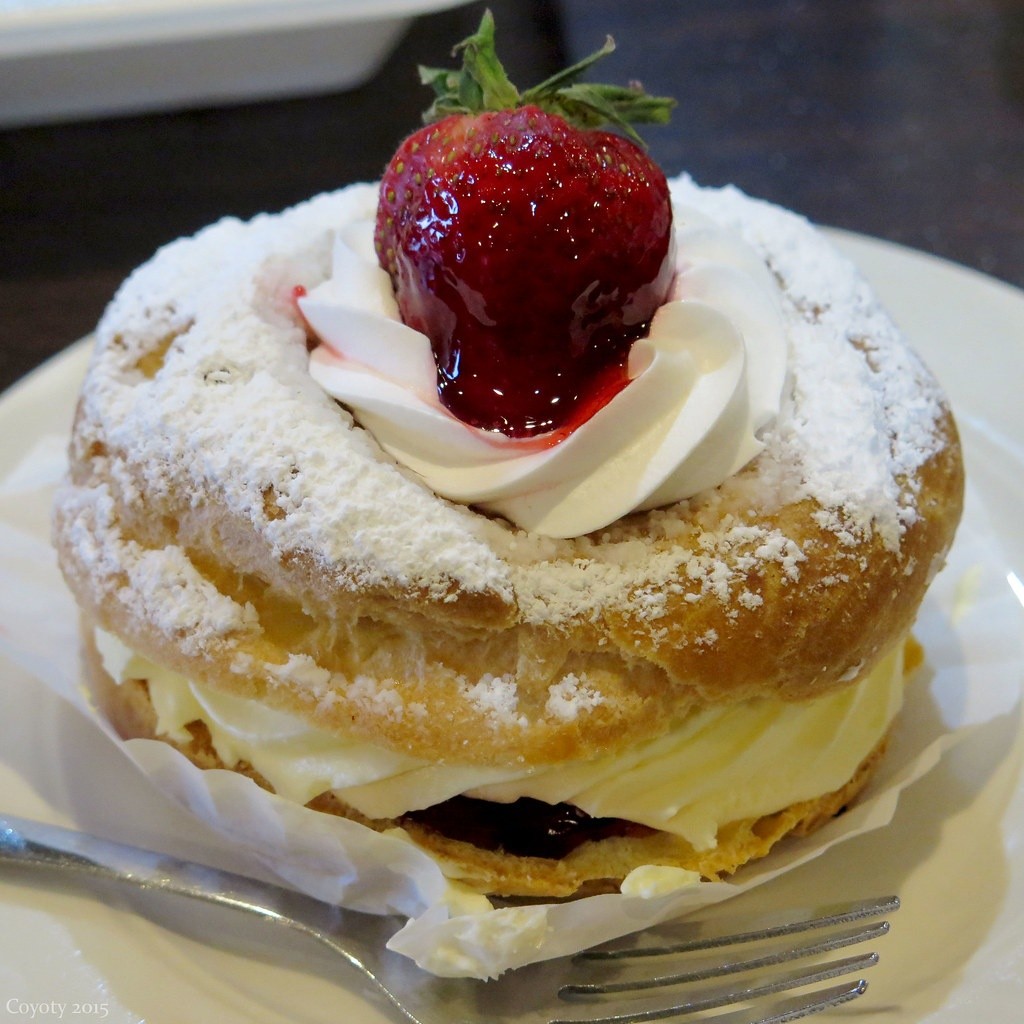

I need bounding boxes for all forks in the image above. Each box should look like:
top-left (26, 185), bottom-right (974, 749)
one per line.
top-left (5, 809), bottom-right (913, 1024)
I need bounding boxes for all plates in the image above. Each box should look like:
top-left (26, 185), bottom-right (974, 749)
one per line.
top-left (0, 216), bottom-right (1024, 1024)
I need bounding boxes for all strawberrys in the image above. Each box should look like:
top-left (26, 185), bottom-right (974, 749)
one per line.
top-left (370, 8), bottom-right (683, 437)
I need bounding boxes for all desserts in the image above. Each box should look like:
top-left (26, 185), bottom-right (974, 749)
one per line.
top-left (58, 174), bottom-right (971, 896)
